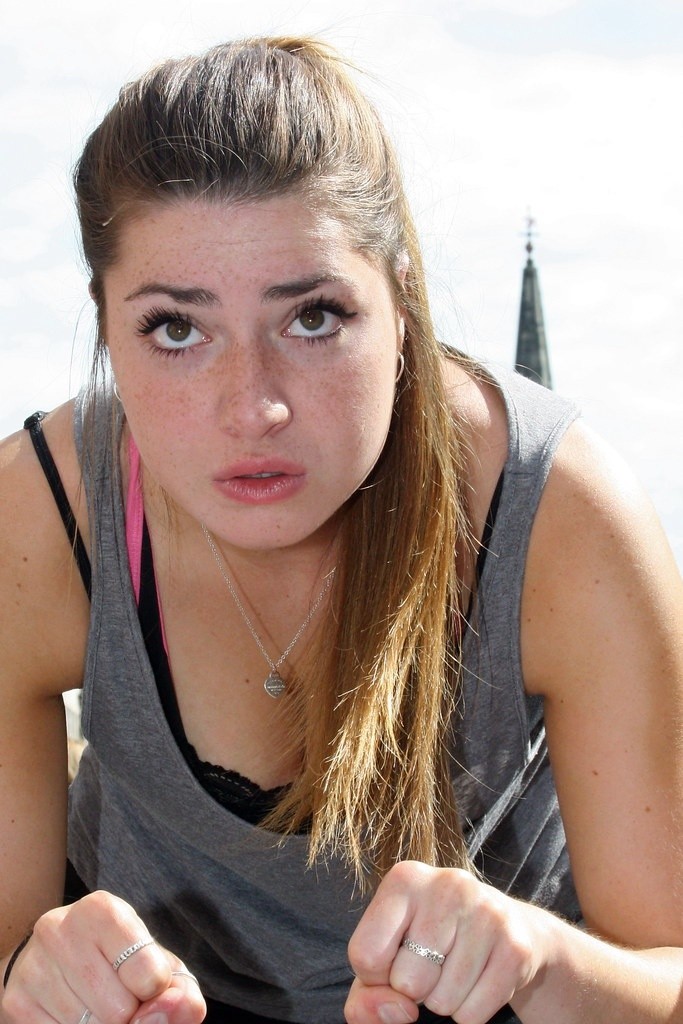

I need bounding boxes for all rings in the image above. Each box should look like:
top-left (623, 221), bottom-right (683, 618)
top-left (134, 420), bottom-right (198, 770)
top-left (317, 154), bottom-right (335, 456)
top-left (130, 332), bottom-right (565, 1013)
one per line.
top-left (400, 936), bottom-right (446, 967)
top-left (80, 1009), bottom-right (91, 1024)
top-left (170, 970), bottom-right (200, 986)
top-left (112, 938), bottom-right (156, 975)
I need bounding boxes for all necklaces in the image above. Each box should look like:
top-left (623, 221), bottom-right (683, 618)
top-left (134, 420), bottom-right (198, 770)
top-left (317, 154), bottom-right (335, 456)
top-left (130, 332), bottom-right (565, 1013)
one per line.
top-left (199, 521), bottom-right (337, 698)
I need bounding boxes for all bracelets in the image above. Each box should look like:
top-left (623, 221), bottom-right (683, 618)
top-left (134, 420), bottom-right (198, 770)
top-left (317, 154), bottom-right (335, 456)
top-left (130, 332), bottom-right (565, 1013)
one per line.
top-left (2, 929), bottom-right (33, 991)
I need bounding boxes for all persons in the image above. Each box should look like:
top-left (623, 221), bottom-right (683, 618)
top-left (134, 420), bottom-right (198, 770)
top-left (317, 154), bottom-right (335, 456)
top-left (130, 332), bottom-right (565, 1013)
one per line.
top-left (0, 35), bottom-right (683, 1024)
